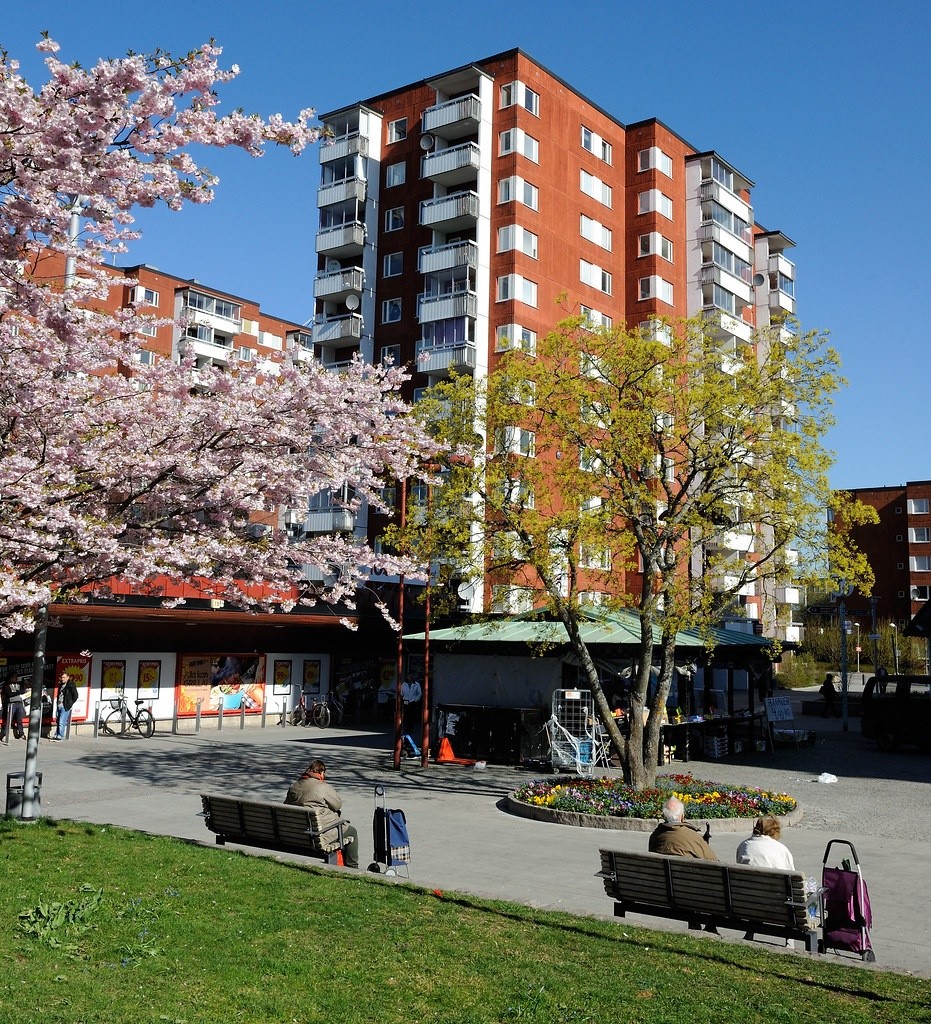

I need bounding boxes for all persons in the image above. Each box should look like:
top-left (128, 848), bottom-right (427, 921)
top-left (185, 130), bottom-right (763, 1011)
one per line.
top-left (402, 673), bottom-right (423, 731)
top-left (735, 814), bottom-right (797, 949)
top-left (49, 672), bottom-right (78, 742)
top-left (648, 796), bottom-right (720, 935)
top-left (283, 760), bottom-right (359, 869)
top-left (876, 665), bottom-right (888, 695)
top-left (2, 672), bottom-right (26, 742)
top-left (821, 674), bottom-right (837, 718)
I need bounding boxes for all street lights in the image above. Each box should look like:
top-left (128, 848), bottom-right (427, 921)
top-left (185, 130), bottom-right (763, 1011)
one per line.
top-left (854, 623), bottom-right (860, 673)
top-left (890, 623), bottom-right (899, 675)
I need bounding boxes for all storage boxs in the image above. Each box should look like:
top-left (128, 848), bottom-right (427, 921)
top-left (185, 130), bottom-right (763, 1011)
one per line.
top-left (704, 728), bottom-right (728, 758)
top-left (754, 739), bottom-right (765, 751)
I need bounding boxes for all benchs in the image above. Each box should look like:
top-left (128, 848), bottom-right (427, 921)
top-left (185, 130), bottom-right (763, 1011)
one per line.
top-left (594, 847), bottom-right (831, 955)
top-left (195, 792), bottom-right (354, 864)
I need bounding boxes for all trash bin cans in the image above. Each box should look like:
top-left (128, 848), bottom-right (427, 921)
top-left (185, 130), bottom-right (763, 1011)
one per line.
top-left (7, 772), bottom-right (42, 818)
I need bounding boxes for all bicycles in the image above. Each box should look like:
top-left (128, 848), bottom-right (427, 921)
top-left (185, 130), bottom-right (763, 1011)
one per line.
top-left (291, 684), bottom-right (330, 729)
top-left (105, 693), bottom-right (155, 738)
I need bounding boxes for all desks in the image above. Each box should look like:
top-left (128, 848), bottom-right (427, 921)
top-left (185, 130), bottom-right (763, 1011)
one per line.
top-left (658, 709), bottom-right (766, 766)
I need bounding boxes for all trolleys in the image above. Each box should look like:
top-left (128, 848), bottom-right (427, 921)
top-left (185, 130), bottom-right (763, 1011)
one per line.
top-left (550, 688), bottom-right (596, 774)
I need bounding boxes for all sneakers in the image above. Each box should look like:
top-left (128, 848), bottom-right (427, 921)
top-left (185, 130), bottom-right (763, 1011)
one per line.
top-left (50, 737), bottom-right (61, 742)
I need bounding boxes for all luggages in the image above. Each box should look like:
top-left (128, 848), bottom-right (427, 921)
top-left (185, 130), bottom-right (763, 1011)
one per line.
top-left (817, 839), bottom-right (876, 962)
top-left (367, 784), bottom-right (412, 879)
